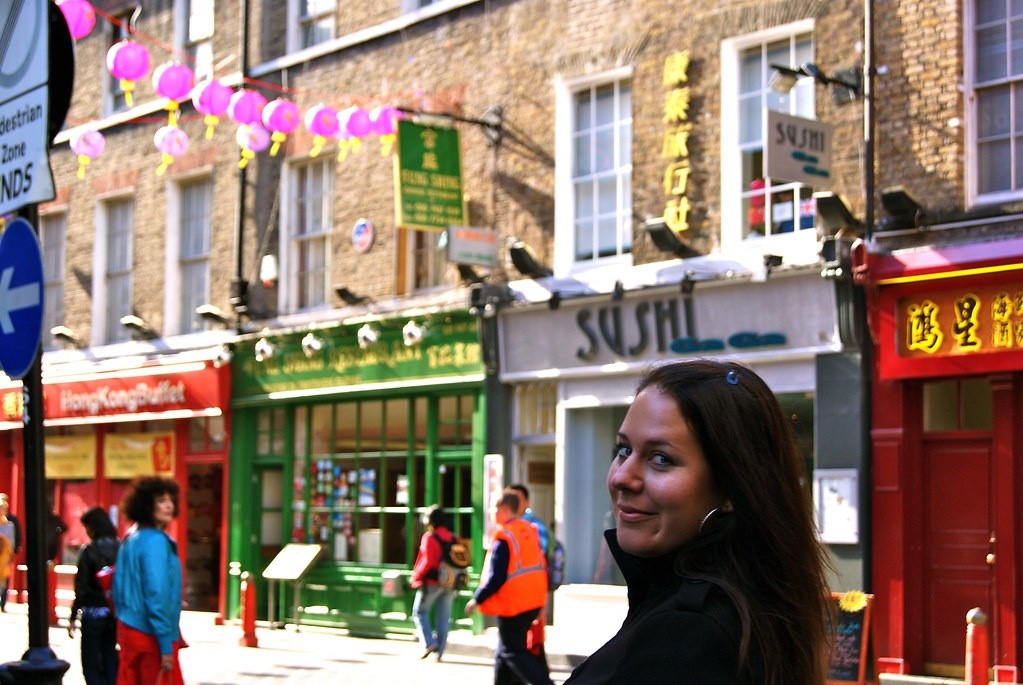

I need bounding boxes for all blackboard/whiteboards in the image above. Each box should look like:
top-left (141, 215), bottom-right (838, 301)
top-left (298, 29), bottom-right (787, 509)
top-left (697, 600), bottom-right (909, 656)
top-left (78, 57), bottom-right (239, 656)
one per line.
top-left (823, 591), bottom-right (880, 685)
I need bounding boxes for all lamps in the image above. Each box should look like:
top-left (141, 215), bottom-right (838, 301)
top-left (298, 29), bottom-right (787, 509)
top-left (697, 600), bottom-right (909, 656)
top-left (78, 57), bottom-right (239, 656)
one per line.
top-left (300, 333), bottom-right (325, 359)
top-left (880, 187), bottom-right (925, 228)
top-left (402, 318), bottom-right (429, 346)
top-left (357, 323), bottom-right (383, 349)
top-left (458, 263), bottom-right (488, 288)
top-left (49, 325), bottom-right (89, 348)
top-left (120, 314), bottom-right (158, 340)
top-left (643, 219), bottom-right (702, 259)
top-left (195, 303), bottom-right (231, 329)
top-left (254, 339), bottom-right (280, 364)
top-left (816, 190), bottom-right (862, 234)
top-left (508, 244), bottom-right (553, 279)
top-left (337, 288), bottom-right (369, 306)
top-left (766, 62), bottom-right (862, 101)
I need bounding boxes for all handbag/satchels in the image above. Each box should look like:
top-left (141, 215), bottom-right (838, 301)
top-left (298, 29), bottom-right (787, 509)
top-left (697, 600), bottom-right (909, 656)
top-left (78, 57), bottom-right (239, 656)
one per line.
top-left (97, 561), bottom-right (117, 613)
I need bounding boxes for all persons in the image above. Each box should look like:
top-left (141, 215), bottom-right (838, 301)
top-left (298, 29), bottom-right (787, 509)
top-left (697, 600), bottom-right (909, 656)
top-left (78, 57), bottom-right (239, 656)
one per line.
top-left (47, 499), bottom-right (67, 626)
top-left (111, 476), bottom-right (189, 685)
top-left (408, 506), bottom-right (453, 661)
top-left (67, 507), bottom-right (120, 685)
top-left (563, 360), bottom-right (834, 685)
top-left (0, 493), bottom-right (22, 613)
top-left (464, 485), bottom-right (556, 685)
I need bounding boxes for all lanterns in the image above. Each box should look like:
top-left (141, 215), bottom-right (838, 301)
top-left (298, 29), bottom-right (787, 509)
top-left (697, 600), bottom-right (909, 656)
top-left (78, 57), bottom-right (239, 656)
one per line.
top-left (304, 103), bottom-right (402, 162)
top-left (234, 125), bottom-right (269, 168)
top-left (153, 126), bottom-right (190, 177)
top-left (69, 127), bottom-right (105, 179)
top-left (106, 38), bottom-right (150, 105)
top-left (54, 0), bottom-right (96, 39)
top-left (191, 79), bottom-right (300, 156)
top-left (151, 60), bottom-right (195, 126)
top-left (748, 177), bottom-right (766, 208)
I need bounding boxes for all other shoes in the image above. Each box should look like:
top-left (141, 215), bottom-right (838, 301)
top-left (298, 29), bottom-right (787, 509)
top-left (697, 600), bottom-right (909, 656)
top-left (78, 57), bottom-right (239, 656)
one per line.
top-left (0, 605), bottom-right (7, 613)
top-left (436, 656), bottom-right (442, 661)
top-left (421, 642), bottom-right (439, 658)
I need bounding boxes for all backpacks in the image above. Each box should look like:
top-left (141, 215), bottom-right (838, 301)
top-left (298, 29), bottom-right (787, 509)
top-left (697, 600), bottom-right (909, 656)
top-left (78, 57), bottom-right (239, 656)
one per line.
top-left (548, 529), bottom-right (564, 592)
top-left (429, 531), bottom-right (469, 589)
